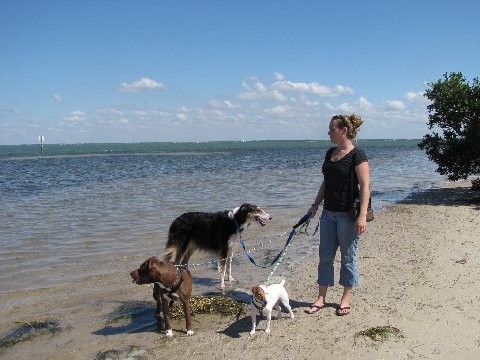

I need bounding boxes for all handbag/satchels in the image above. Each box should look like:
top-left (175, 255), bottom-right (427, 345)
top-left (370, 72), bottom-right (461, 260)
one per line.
top-left (351, 203), bottom-right (374, 222)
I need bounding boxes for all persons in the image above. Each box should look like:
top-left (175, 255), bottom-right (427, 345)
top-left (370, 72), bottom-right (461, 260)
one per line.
top-left (304, 114), bottom-right (370, 316)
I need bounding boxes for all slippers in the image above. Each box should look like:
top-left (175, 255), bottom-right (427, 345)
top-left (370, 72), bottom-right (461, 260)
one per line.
top-left (336, 304), bottom-right (351, 316)
top-left (303, 302), bottom-right (325, 313)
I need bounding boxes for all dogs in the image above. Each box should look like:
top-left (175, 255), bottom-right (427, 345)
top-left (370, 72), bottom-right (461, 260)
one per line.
top-left (161, 202), bottom-right (274, 290)
top-left (129, 257), bottom-right (196, 339)
top-left (249, 278), bottom-right (295, 335)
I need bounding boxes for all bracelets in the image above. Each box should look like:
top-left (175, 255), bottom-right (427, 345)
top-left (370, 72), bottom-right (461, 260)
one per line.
top-left (312, 203), bottom-right (318, 206)
